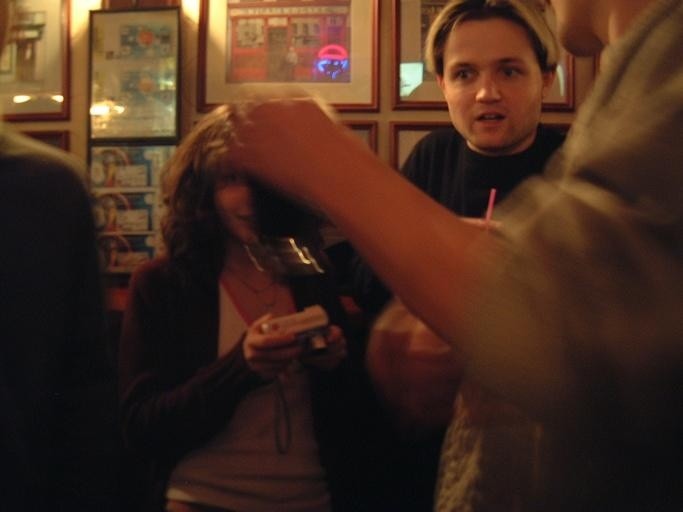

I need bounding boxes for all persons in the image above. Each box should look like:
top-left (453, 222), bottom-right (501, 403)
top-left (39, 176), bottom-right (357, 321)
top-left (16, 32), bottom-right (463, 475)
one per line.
top-left (2, 0), bottom-right (681, 511)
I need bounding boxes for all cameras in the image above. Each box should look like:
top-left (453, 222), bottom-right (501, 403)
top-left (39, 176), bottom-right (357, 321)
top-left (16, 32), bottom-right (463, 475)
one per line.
top-left (259, 305), bottom-right (331, 371)
